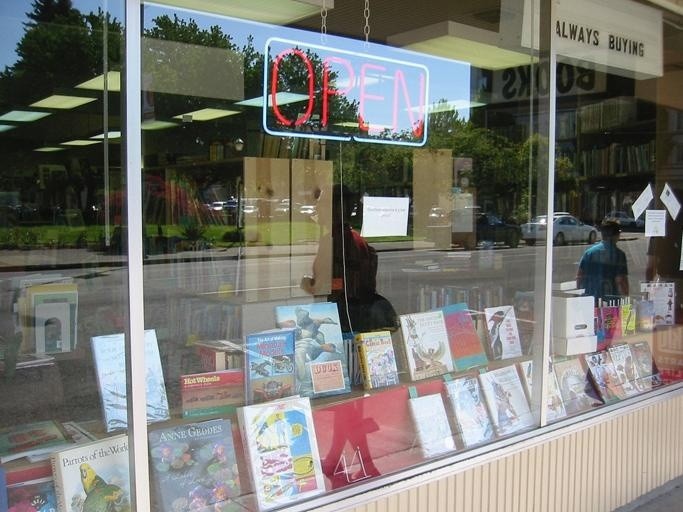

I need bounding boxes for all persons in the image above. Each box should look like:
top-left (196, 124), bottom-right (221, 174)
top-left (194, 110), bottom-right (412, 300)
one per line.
top-left (306, 182), bottom-right (380, 481)
top-left (575, 216), bottom-right (631, 405)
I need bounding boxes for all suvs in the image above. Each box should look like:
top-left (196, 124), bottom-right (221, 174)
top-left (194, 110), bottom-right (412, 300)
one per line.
top-left (422, 207), bottom-right (522, 251)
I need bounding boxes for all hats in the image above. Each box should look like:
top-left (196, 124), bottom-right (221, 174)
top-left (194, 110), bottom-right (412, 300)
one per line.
top-left (332, 183), bottom-right (361, 199)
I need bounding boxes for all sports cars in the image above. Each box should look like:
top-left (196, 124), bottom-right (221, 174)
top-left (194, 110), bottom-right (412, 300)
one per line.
top-left (252, 380), bottom-right (291, 404)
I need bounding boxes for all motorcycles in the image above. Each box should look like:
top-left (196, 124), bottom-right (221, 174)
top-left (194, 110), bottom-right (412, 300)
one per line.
top-left (274, 355), bottom-right (292, 373)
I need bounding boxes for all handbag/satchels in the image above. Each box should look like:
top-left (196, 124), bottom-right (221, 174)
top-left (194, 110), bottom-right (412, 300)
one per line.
top-left (352, 288), bottom-right (402, 339)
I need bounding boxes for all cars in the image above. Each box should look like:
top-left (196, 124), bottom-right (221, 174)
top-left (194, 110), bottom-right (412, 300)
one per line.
top-left (520, 212), bottom-right (598, 247)
top-left (203, 196), bottom-right (481, 226)
top-left (602, 210), bottom-right (644, 231)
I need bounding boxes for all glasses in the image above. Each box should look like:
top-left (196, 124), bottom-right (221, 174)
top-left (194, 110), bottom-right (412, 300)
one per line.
top-left (618, 227), bottom-right (623, 233)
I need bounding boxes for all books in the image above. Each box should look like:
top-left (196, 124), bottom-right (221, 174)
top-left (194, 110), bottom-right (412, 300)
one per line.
top-left (1, 278), bottom-right (326, 510)
top-left (275, 277), bottom-right (567, 460)
top-left (553, 279), bottom-right (681, 416)
top-left (556, 98), bottom-right (656, 224)
